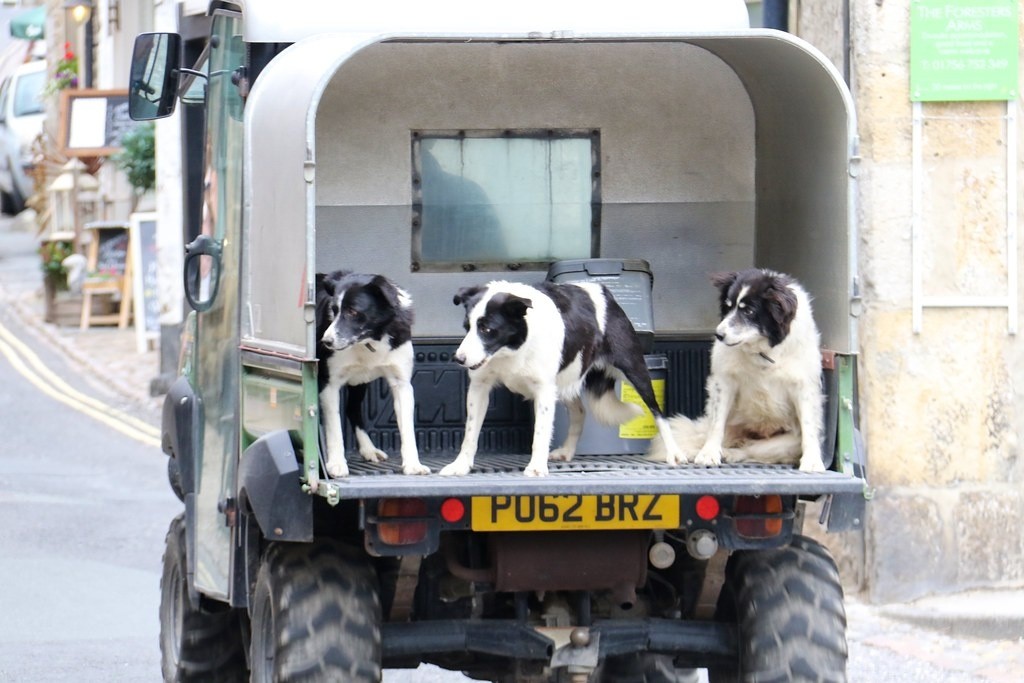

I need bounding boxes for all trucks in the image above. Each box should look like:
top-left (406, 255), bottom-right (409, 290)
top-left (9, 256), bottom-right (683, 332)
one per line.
top-left (126, 0), bottom-right (870, 683)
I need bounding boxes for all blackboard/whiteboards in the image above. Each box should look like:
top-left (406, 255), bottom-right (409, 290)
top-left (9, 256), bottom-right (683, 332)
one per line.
top-left (132, 211), bottom-right (166, 340)
top-left (82, 225), bottom-right (134, 290)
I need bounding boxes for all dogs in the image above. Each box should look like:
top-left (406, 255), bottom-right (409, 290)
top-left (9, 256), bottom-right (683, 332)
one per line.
top-left (314, 270), bottom-right (432, 477)
top-left (650, 268), bottom-right (828, 474)
top-left (433, 279), bottom-right (690, 477)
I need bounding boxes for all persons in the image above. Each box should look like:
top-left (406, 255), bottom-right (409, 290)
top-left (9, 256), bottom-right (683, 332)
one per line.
top-left (419, 133), bottom-right (506, 263)
top-left (129, 33), bottom-right (157, 92)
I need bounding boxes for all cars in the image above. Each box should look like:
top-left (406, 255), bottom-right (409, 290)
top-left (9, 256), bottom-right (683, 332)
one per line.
top-left (0, 61), bottom-right (48, 215)
